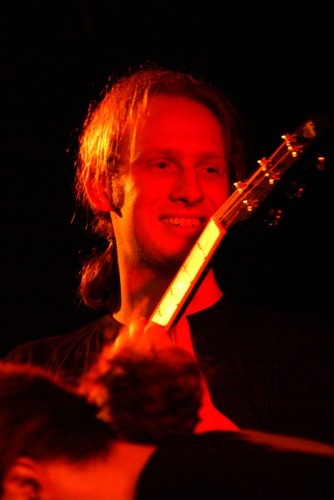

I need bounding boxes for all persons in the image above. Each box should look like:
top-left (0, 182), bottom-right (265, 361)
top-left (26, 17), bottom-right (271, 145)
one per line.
top-left (0, 360), bottom-right (334, 500)
top-left (0, 67), bottom-right (334, 457)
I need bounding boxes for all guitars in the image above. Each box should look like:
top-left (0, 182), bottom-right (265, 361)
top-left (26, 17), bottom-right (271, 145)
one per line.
top-left (150, 116), bottom-right (327, 334)
